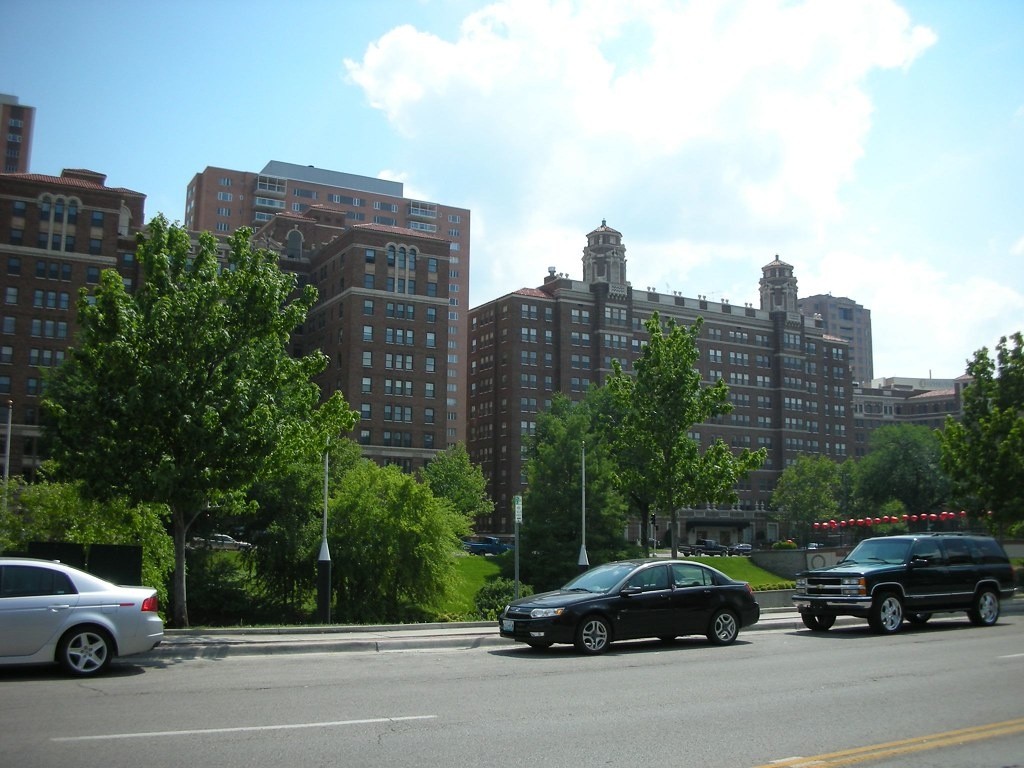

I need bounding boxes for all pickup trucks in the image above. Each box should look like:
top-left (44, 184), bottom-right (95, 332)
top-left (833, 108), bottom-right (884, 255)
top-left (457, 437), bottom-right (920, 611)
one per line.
top-left (678, 538), bottom-right (728, 557)
top-left (463, 537), bottom-right (514, 556)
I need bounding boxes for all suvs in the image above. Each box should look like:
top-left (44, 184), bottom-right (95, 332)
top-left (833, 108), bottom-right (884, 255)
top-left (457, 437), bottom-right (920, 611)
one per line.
top-left (791, 532), bottom-right (1017, 635)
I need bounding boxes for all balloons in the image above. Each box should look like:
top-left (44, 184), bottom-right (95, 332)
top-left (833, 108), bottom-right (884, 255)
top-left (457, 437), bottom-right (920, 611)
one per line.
top-left (988, 511), bottom-right (992, 515)
top-left (813, 511), bottom-right (966, 528)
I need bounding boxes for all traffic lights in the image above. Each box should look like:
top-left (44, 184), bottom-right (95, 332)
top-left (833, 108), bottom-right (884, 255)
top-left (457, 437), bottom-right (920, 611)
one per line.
top-left (650, 513), bottom-right (655, 524)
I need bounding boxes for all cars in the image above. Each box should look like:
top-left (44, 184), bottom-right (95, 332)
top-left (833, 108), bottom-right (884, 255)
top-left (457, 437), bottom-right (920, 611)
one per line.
top-left (802, 542), bottom-right (824, 549)
top-left (727, 543), bottom-right (751, 556)
top-left (498, 558), bottom-right (760, 655)
top-left (191, 534), bottom-right (251, 551)
top-left (0, 556), bottom-right (164, 678)
